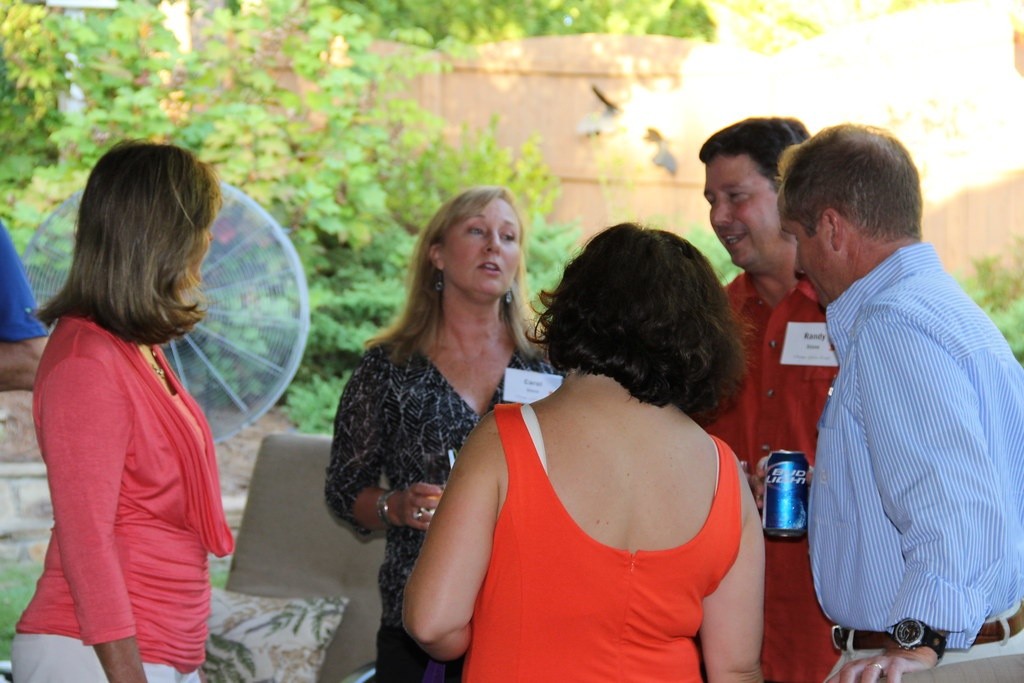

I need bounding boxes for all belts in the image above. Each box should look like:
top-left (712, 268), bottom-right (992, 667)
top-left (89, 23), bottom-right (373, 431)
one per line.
top-left (834, 596), bottom-right (1023, 650)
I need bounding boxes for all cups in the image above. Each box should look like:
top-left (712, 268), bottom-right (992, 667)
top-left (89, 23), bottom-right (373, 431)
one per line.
top-left (418, 449), bottom-right (456, 520)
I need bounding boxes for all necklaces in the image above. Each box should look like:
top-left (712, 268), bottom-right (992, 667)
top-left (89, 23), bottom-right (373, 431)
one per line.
top-left (138, 343), bottom-right (164, 375)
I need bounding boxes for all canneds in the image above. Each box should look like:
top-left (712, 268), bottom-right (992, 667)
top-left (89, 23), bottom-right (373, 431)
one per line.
top-left (761, 449), bottom-right (809, 537)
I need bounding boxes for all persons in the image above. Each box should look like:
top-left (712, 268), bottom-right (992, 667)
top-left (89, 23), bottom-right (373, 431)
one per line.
top-left (0, 220), bottom-right (50, 392)
top-left (692, 116), bottom-right (842, 683)
top-left (401, 223), bottom-right (766, 683)
top-left (324, 186), bottom-right (578, 683)
top-left (11, 139), bottom-right (235, 683)
top-left (750, 123), bottom-right (1024, 683)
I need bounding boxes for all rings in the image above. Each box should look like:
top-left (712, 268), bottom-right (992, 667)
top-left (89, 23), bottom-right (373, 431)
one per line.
top-left (867, 663), bottom-right (883, 678)
top-left (417, 508), bottom-right (423, 520)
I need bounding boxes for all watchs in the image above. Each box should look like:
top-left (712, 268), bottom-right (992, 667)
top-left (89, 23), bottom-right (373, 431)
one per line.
top-left (376, 490), bottom-right (404, 528)
top-left (891, 618), bottom-right (947, 658)
